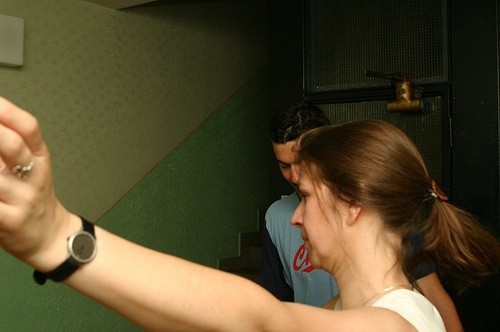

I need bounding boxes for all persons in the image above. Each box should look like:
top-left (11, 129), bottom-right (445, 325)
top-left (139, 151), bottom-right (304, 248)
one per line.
top-left (264, 99), bottom-right (466, 332)
top-left (0, 97), bottom-right (500, 332)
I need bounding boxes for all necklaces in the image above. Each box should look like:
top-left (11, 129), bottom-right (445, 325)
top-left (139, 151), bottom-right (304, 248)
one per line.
top-left (363, 284), bottom-right (412, 306)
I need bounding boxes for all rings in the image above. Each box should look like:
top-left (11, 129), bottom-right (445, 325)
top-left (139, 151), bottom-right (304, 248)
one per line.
top-left (13, 161), bottom-right (34, 178)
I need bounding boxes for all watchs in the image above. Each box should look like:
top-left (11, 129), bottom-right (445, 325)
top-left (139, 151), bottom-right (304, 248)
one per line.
top-left (33, 216), bottom-right (97, 285)
